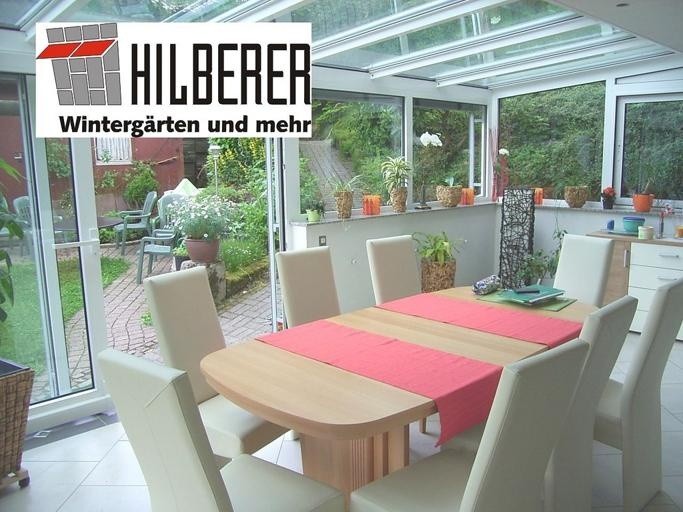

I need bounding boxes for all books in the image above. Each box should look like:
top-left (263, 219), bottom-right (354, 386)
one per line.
top-left (499, 284), bottom-right (566, 306)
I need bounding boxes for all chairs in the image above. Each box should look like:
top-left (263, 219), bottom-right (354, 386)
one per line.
top-left (117, 190), bottom-right (181, 283)
top-left (12, 191), bottom-right (63, 261)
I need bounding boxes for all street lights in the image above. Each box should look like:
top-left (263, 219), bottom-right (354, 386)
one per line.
top-left (209, 143), bottom-right (221, 203)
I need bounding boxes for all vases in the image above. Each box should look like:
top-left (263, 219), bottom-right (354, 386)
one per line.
top-left (182, 236), bottom-right (221, 264)
top-left (301, 156), bottom-right (474, 223)
top-left (526, 184), bottom-right (667, 241)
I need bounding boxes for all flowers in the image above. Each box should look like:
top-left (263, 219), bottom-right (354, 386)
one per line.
top-left (161, 192), bottom-right (246, 239)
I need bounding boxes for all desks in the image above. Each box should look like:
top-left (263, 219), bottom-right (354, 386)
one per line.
top-left (50, 212), bottom-right (124, 238)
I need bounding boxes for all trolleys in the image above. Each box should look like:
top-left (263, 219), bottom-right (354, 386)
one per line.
top-left (0, 467), bottom-right (30, 493)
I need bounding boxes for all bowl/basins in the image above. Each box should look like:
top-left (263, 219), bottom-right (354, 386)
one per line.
top-left (623, 216), bottom-right (644, 231)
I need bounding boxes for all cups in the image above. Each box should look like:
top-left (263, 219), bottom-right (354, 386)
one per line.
top-left (530, 188), bottom-right (543, 205)
top-left (362, 194), bottom-right (379, 215)
top-left (675, 226), bottom-right (682, 238)
top-left (637, 226), bottom-right (653, 239)
top-left (458, 189), bottom-right (474, 205)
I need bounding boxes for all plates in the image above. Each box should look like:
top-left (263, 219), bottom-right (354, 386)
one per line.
top-left (610, 230), bottom-right (638, 236)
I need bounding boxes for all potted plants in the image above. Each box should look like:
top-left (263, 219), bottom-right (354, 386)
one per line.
top-left (0, 155), bottom-right (35, 491)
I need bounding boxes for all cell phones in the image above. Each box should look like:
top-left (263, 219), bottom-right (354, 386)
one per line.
top-left (515, 288), bottom-right (539, 293)
